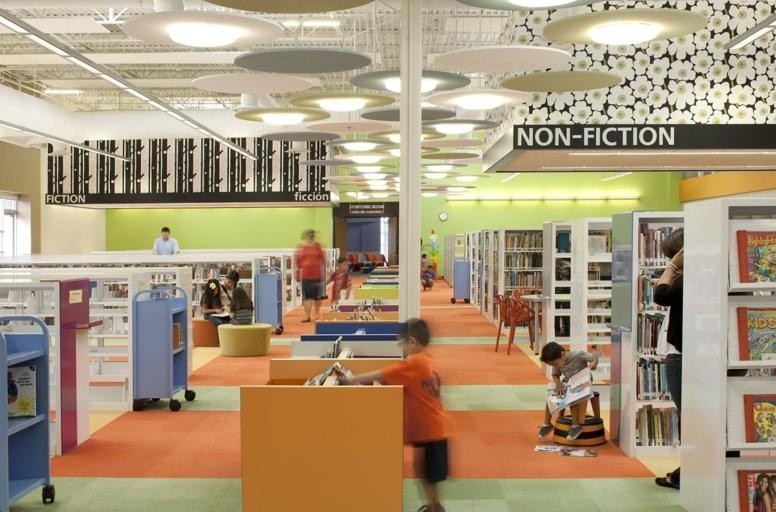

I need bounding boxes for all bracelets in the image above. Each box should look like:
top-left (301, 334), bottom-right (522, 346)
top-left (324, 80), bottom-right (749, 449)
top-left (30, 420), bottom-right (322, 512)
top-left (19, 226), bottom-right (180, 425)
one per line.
top-left (668, 261), bottom-right (681, 274)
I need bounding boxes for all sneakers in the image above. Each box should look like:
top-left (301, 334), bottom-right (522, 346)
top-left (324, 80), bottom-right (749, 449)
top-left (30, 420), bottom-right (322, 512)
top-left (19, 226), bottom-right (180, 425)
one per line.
top-left (537, 423), bottom-right (553, 439)
top-left (566, 424), bottom-right (585, 441)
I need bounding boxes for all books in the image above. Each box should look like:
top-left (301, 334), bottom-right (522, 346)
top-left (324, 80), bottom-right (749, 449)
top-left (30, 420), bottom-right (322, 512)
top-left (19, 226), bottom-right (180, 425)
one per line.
top-left (637, 224), bottom-right (683, 447)
top-left (535, 444), bottom-right (596, 457)
top-left (95, 255), bottom-right (301, 326)
top-left (742, 392), bottom-right (776, 443)
top-left (465, 231), bottom-right (544, 326)
top-left (738, 469), bottom-right (776, 511)
top-left (7, 362), bottom-right (39, 419)
top-left (736, 305), bottom-right (776, 360)
top-left (736, 228), bottom-right (776, 284)
top-left (300, 336), bottom-right (356, 385)
top-left (547, 366), bottom-right (595, 417)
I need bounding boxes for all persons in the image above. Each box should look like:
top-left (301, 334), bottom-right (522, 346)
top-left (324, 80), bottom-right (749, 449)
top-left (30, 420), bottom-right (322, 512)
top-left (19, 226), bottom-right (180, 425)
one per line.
top-left (296, 229), bottom-right (328, 324)
top-left (339, 317), bottom-right (451, 512)
top-left (422, 254), bottom-right (428, 270)
top-left (420, 265), bottom-right (435, 291)
top-left (326, 255), bottom-right (352, 309)
top-left (752, 473), bottom-right (775, 511)
top-left (654, 227), bottom-right (684, 489)
top-left (536, 342), bottom-right (599, 443)
top-left (153, 226), bottom-right (180, 256)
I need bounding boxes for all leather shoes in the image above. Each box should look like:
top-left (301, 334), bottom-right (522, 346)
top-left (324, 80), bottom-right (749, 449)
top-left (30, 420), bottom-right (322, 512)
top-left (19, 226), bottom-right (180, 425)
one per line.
top-left (301, 318), bottom-right (311, 322)
top-left (655, 472), bottom-right (679, 490)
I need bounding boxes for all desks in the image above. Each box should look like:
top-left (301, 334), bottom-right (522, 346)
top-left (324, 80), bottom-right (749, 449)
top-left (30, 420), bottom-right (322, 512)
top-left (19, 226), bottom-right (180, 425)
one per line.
top-left (243, 271), bottom-right (405, 512)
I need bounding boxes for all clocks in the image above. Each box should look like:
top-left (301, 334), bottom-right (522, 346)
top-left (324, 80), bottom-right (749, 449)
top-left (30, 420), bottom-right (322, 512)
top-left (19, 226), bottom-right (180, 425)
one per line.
top-left (439, 211), bottom-right (448, 221)
